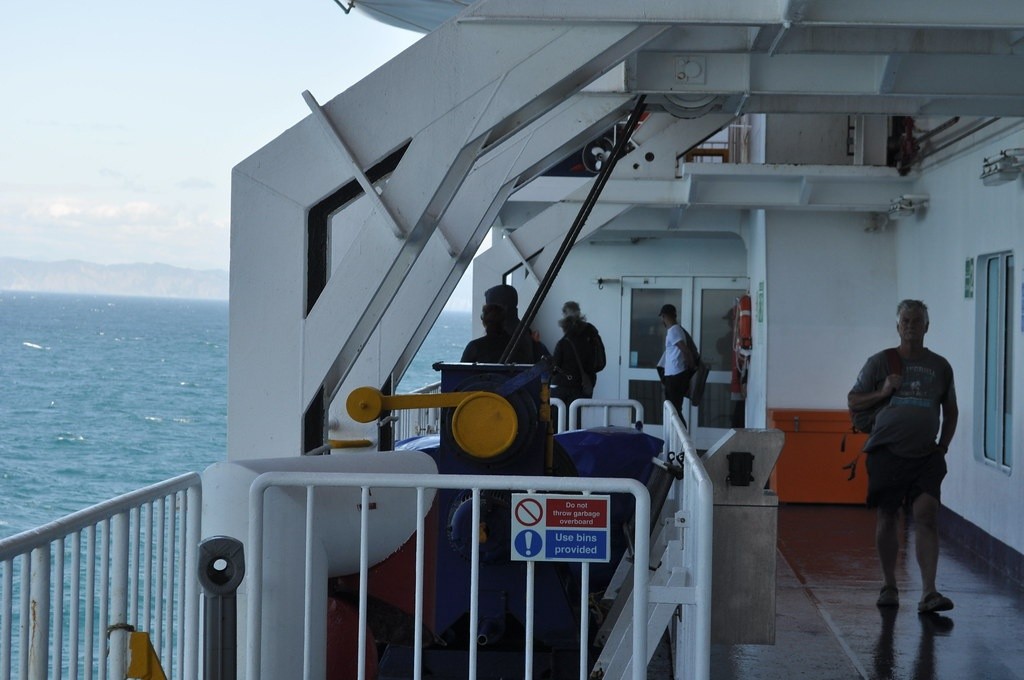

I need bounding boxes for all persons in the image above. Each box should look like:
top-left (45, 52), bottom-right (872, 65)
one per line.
top-left (657, 304), bottom-right (701, 428)
top-left (461, 284), bottom-right (552, 365)
top-left (551, 300), bottom-right (607, 399)
top-left (847, 299), bottom-right (959, 611)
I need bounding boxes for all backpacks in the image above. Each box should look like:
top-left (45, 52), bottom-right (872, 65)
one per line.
top-left (588, 333), bottom-right (607, 374)
top-left (851, 348), bottom-right (903, 434)
top-left (548, 368), bottom-right (584, 405)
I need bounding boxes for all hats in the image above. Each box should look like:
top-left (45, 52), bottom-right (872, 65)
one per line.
top-left (658, 303), bottom-right (676, 317)
top-left (486, 284), bottom-right (519, 309)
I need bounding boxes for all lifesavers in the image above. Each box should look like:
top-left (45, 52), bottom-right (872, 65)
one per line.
top-left (733, 294), bottom-right (752, 361)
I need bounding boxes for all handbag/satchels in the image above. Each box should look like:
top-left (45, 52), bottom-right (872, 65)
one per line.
top-left (684, 367), bottom-right (711, 406)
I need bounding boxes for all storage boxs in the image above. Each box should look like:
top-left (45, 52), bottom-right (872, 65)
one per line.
top-left (767, 405), bottom-right (871, 504)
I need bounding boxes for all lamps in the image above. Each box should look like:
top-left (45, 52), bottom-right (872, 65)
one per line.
top-left (977, 147), bottom-right (1024, 187)
top-left (886, 192), bottom-right (928, 221)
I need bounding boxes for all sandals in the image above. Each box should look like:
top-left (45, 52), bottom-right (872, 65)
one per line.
top-left (918, 592), bottom-right (954, 612)
top-left (876, 584), bottom-right (900, 605)
